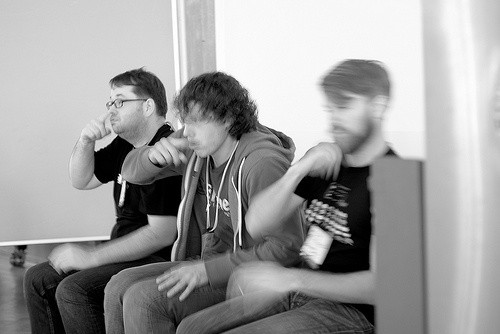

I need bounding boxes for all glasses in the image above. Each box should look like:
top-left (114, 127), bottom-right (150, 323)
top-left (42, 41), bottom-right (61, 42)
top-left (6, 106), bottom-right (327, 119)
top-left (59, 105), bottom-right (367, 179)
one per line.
top-left (106, 99), bottom-right (147, 110)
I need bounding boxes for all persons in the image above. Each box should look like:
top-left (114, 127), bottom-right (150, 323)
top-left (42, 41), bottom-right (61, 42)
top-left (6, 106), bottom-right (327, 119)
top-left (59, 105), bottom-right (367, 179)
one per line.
top-left (23, 68), bottom-right (202, 334)
top-left (176, 60), bottom-right (402, 334)
top-left (103, 72), bottom-right (306, 334)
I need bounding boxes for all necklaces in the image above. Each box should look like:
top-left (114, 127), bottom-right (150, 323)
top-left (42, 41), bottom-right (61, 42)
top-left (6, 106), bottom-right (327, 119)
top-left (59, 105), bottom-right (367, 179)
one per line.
top-left (117, 122), bottom-right (176, 184)
